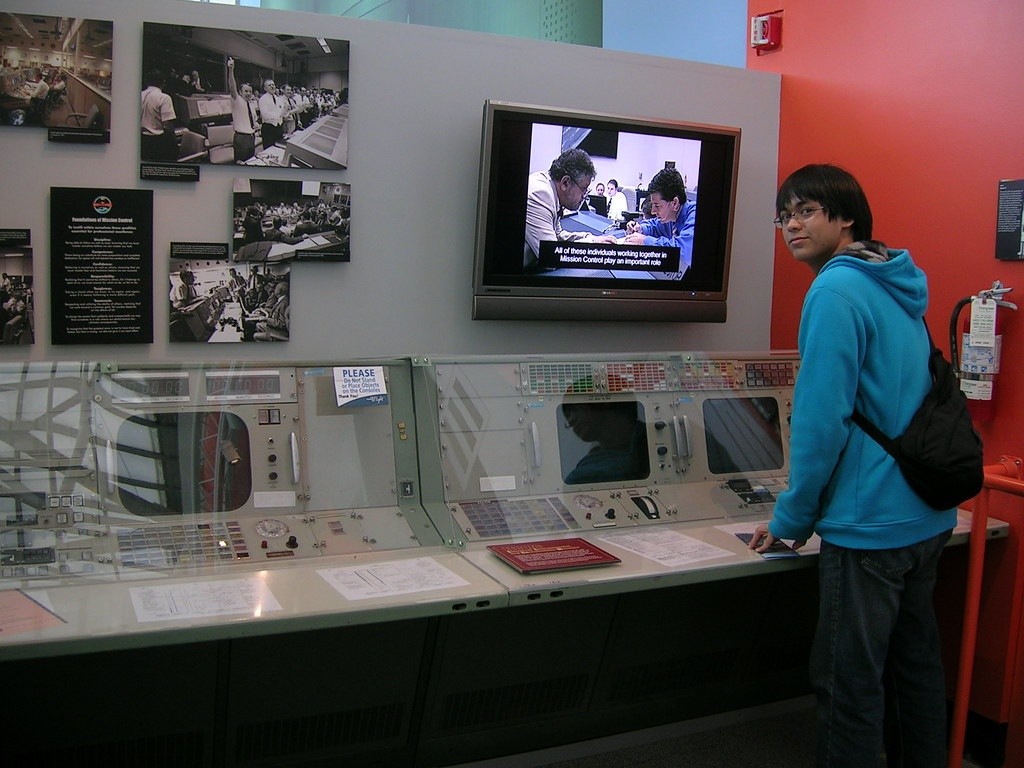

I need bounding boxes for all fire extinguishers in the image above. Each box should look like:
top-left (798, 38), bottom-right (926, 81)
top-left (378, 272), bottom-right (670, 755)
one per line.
top-left (949, 279), bottom-right (1018, 422)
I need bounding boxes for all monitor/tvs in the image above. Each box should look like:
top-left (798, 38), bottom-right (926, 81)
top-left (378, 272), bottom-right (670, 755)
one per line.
top-left (471, 99), bottom-right (742, 323)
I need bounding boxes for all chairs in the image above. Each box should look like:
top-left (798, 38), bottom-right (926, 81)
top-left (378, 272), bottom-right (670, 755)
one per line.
top-left (619, 187), bottom-right (636, 211)
top-left (57, 103), bottom-right (100, 128)
top-left (176, 130), bottom-right (209, 163)
top-left (0, 303), bottom-right (34, 345)
top-left (207, 125), bottom-right (234, 164)
top-left (36, 75), bottom-right (67, 118)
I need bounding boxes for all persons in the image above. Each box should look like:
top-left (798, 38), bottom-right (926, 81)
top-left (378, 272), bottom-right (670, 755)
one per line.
top-left (140, 57), bottom-right (350, 342)
top-left (562, 376), bottom-right (649, 486)
top-left (747, 163), bottom-right (958, 768)
top-left (0, 62), bottom-right (111, 127)
top-left (0, 272), bottom-right (33, 344)
top-left (523, 149), bottom-right (697, 275)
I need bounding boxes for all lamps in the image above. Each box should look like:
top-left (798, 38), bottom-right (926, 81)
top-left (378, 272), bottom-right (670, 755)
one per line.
top-left (219, 439), bottom-right (240, 465)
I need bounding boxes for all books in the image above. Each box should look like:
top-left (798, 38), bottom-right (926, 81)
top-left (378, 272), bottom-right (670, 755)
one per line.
top-left (486, 537), bottom-right (622, 574)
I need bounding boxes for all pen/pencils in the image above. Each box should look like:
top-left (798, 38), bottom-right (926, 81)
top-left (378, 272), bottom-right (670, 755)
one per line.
top-left (634, 221), bottom-right (636, 233)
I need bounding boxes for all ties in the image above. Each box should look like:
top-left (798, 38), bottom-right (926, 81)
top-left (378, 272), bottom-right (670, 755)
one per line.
top-left (246, 100), bottom-right (255, 129)
top-left (272, 94), bottom-right (276, 105)
top-left (187, 287), bottom-right (190, 297)
top-left (253, 275), bottom-right (255, 288)
top-left (287, 98), bottom-right (294, 119)
top-left (302, 95), bottom-right (303, 102)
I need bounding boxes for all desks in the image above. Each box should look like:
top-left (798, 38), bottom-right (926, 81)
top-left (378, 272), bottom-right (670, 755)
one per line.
top-left (233, 215), bottom-right (346, 261)
top-left (560, 210), bottom-right (626, 239)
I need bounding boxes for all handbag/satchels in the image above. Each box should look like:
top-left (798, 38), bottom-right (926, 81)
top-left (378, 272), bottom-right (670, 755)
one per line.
top-left (894, 350), bottom-right (983, 510)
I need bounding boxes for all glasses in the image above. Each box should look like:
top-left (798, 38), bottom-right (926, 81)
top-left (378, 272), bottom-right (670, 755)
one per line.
top-left (564, 405), bottom-right (599, 428)
top-left (773, 207), bottom-right (829, 228)
top-left (567, 174), bottom-right (591, 198)
